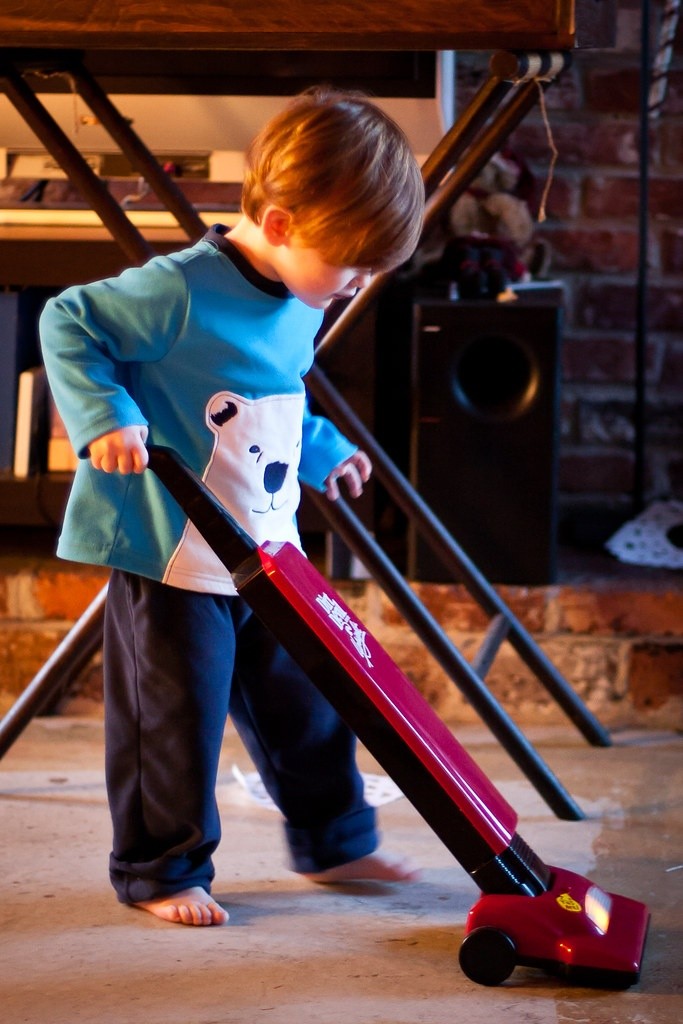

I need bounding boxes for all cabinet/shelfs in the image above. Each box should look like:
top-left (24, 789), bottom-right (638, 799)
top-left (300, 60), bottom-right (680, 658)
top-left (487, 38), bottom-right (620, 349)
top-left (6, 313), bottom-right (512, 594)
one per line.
top-left (1, 145), bottom-right (381, 585)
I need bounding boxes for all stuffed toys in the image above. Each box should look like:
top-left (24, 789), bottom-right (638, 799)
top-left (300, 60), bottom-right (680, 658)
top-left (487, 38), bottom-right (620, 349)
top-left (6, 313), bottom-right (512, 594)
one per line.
top-left (447, 149), bottom-right (536, 299)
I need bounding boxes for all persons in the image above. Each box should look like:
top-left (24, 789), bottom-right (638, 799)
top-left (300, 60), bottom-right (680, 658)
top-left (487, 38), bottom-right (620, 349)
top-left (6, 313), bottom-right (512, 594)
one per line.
top-left (31, 85), bottom-right (424, 924)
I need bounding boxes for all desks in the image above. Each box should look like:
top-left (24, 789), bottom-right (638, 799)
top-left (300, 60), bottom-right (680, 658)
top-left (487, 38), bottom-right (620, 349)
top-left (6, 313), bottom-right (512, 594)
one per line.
top-left (1, 1), bottom-right (619, 828)
